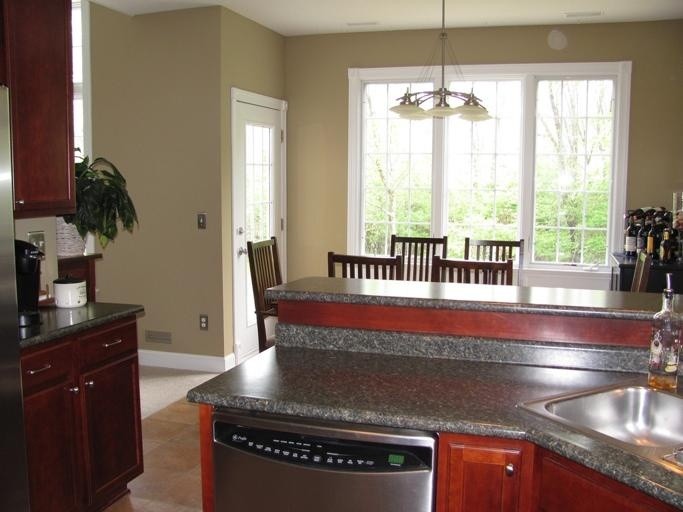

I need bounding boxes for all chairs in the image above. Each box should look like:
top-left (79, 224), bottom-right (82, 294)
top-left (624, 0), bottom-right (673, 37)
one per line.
top-left (328, 252), bottom-right (402, 280)
top-left (431, 256), bottom-right (513, 284)
top-left (630, 249), bottom-right (652, 292)
top-left (464, 238), bottom-right (524, 285)
top-left (391, 235), bottom-right (447, 282)
top-left (247, 237), bottom-right (282, 353)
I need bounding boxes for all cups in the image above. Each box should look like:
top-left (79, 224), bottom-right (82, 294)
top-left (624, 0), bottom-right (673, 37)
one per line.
top-left (53, 279), bottom-right (87, 307)
top-left (55, 308), bottom-right (88, 329)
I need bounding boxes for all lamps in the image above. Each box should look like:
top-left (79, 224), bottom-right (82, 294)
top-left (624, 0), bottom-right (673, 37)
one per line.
top-left (389, 0), bottom-right (491, 121)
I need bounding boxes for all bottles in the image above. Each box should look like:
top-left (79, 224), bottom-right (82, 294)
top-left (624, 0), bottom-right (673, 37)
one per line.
top-left (623, 206), bottom-right (677, 264)
top-left (20, 312), bottom-right (26, 326)
top-left (648, 289), bottom-right (683, 391)
top-left (20, 328), bottom-right (27, 339)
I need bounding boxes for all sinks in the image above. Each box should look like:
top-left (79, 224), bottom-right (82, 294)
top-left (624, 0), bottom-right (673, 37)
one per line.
top-left (511, 374), bottom-right (682, 475)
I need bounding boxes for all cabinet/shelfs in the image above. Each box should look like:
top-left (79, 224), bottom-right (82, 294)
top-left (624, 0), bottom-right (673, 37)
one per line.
top-left (435, 430), bottom-right (528, 512)
top-left (609, 253), bottom-right (683, 293)
top-left (19, 315), bottom-right (146, 511)
top-left (527, 441), bottom-right (681, 512)
top-left (57, 254), bottom-right (104, 303)
top-left (0, 0), bottom-right (78, 219)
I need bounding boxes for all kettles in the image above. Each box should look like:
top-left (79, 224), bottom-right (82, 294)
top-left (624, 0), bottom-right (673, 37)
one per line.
top-left (14, 239), bottom-right (45, 325)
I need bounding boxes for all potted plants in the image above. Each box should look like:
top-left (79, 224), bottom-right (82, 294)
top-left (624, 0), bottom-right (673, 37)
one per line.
top-left (58, 146), bottom-right (139, 257)
